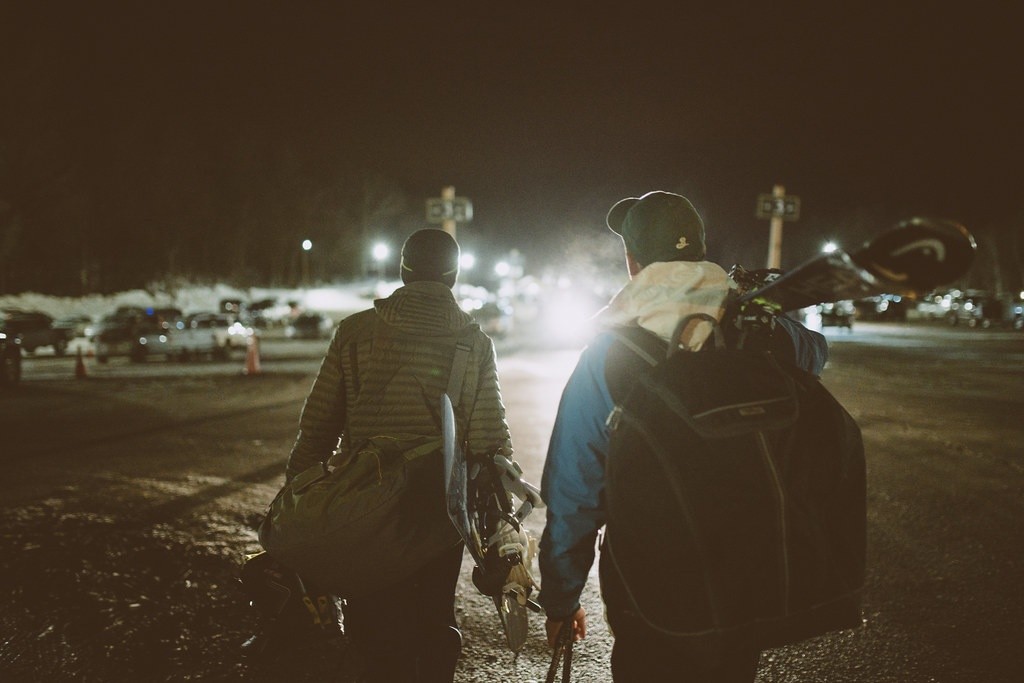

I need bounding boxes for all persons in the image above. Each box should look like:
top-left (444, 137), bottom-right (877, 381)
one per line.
top-left (283, 229), bottom-right (516, 682)
top-left (540, 192), bottom-right (830, 683)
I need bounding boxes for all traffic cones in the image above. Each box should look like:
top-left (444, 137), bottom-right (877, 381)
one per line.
top-left (243, 334), bottom-right (262, 374)
top-left (72, 345), bottom-right (86, 375)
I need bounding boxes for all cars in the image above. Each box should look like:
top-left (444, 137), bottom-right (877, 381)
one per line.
top-left (800, 286), bottom-right (1024, 330)
top-left (0, 283), bottom-right (532, 361)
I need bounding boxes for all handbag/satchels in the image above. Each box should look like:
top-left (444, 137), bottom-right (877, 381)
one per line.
top-left (256, 432), bottom-right (416, 584)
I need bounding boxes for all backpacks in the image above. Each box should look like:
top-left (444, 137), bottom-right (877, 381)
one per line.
top-left (598, 290), bottom-right (868, 655)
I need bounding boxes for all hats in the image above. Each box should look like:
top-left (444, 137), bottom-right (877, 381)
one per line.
top-left (606, 190), bottom-right (708, 267)
top-left (399, 228), bottom-right (460, 288)
